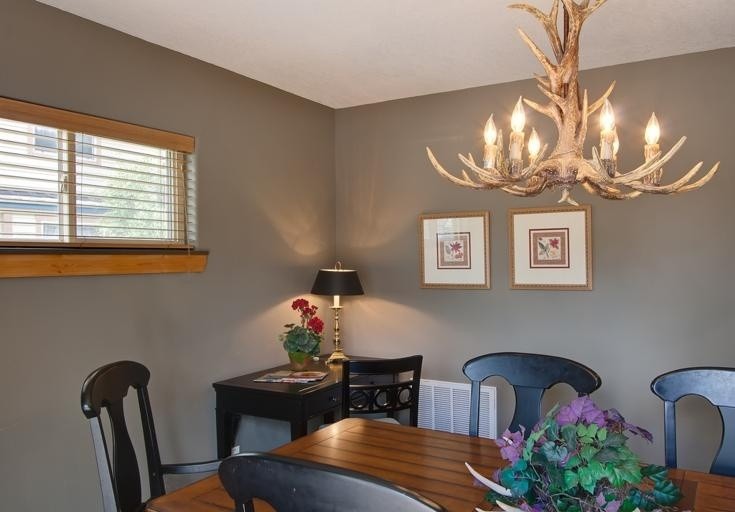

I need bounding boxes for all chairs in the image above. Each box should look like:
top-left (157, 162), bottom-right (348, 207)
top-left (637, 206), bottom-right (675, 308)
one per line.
top-left (80, 360), bottom-right (222, 511)
top-left (462, 351), bottom-right (602, 440)
top-left (342, 355), bottom-right (423, 427)
top-left (650, 366), bottom-right (735, 476)
top-left (218, 452), bottom-right (448, 511)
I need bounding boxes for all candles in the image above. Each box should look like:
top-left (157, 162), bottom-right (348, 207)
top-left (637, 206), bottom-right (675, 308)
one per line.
top-left (510, 96), bottom-right (527, 160)
top-left (482, 113), bottom-right (497, 168)
top-left (643, 112), bottom-right (661, 185)
top-left (599, 97), bottom-right (620, 159)
top-left (527, 126), bottom-right (541, 162)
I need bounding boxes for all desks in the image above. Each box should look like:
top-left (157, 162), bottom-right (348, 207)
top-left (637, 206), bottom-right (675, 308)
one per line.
top-left (143, 417), bottom-right (733, 511)
top-left (212, 354), bottom-right (399, 460)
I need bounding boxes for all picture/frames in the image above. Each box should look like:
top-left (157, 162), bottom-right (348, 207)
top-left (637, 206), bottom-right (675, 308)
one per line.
top-left (507, 205), bottom-right (593, 290)
top-left (419, 211), bottom-right (491, 289)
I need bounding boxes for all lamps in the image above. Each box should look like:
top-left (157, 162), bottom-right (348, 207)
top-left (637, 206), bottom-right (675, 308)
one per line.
top-left (310, 261), bottom-right (365, 364)
